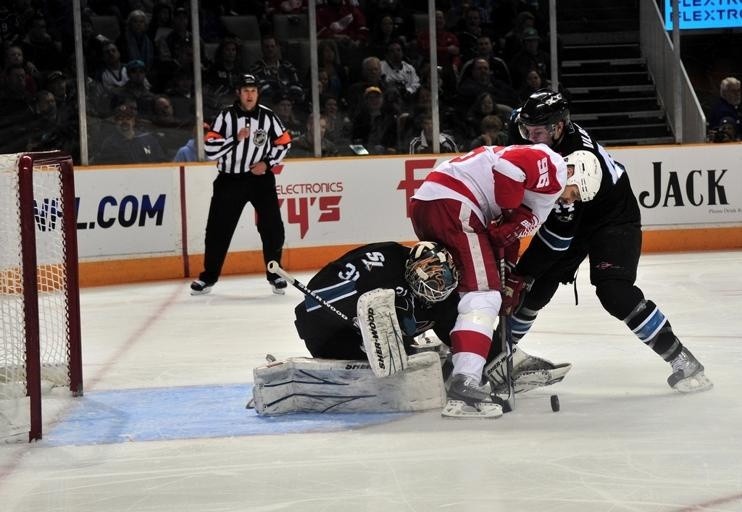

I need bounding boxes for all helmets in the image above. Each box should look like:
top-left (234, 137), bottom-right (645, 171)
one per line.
top-left (561, 150), bottom-right (603, 203)
top-left (234, 73), bottom-right (262, 89)
top-left (404, 241), bottom-right (462, 304)
top-left (513, 88), bottom-right (569, 148)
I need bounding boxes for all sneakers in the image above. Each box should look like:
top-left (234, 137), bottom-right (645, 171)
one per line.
top-left (191, 278), bottom-right (209, 292)
top-left (273, 277), bottom-right (288, 288)
top-left (667, 346), bottom-right (705, 387)
top-left (442, 353), bottom-right (492, 403)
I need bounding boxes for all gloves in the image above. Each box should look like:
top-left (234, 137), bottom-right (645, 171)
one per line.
top-left (487, 207), bottom-right (541, 248)
top-left (498, 265), bottom-right (528, 316)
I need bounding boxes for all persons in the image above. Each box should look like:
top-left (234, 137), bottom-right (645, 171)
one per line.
top-left (190, 74), bottom-right (292, 296)
top-left (656, 0), bottom-right (742, 144)
top-left (0, 0), bottom-right (82, 166)
top-left (296, 239), bottom-right (572, 396)
top-left (408, 144), bottom-right (602, 420)
top-left (79, 2), bottom-right (552, 166)
top-left (497, 89), bottom-right (713, 393)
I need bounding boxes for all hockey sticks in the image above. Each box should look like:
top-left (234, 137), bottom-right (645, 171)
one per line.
top-left (491, 245), bottom-right (516, 413)
